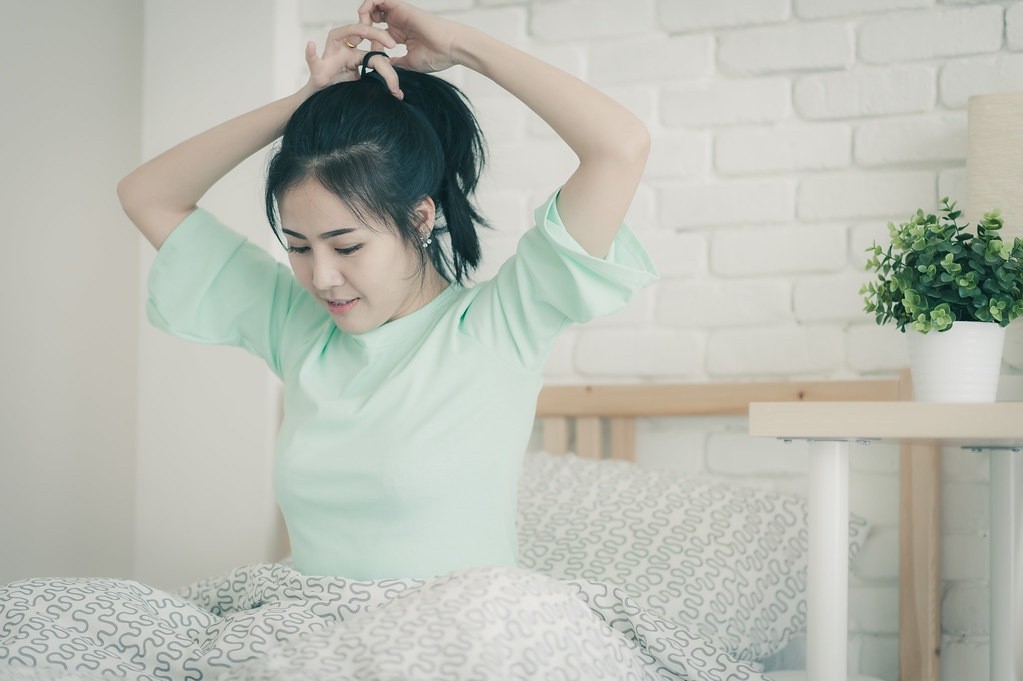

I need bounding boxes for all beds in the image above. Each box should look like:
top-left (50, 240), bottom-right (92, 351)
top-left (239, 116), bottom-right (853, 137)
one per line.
top-left (4, 370), bottom-right (922, 681)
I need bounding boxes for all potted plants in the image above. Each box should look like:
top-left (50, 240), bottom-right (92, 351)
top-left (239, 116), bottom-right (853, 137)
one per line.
top-left (860, 198), bottom-right (1023, 404)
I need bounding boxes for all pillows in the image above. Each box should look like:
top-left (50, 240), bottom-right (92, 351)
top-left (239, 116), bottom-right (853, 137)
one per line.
top-left (512, 456), bottom-right (872, 660)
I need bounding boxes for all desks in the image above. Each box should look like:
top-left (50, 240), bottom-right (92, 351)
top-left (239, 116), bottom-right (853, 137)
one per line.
top-left (759, 395), bottom-right (1023, 681)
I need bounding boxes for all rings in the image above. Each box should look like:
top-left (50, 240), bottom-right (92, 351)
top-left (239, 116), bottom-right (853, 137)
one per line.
top-left (343, 38), bottom-right (355, 49)
top-left (380, 11), bottom-right (385, 22)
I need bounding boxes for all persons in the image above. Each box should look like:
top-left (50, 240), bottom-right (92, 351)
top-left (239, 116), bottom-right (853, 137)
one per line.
top-left (118, 0), bottom-right (661, 582)
